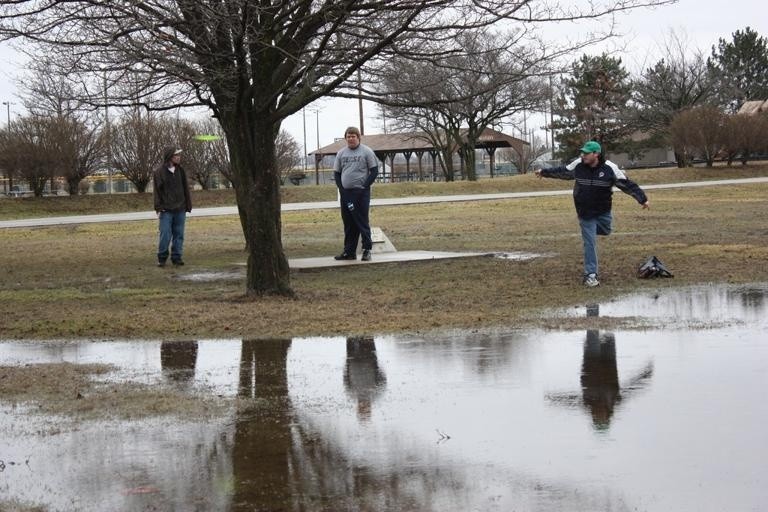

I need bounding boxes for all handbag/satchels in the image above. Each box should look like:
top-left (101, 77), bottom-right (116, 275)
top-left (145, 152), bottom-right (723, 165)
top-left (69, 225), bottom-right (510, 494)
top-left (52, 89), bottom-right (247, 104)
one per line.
top-left (637, 254), bottom-right (674, 279)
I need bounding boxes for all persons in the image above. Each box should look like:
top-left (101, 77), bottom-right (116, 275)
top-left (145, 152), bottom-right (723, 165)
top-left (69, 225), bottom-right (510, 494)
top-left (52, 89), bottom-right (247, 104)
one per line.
top-left (332, 127), bottom-right (379, 261)
top-left (160, 342), bottom-right (200, 381)
top-left (154, 146), bottom-right (192, 266)
top-left (535, 140), bottom-right (650, 290)
top-left (541, 303), bottom-right (659, 435)
top-left (341, 335), bottom-right (387, 423)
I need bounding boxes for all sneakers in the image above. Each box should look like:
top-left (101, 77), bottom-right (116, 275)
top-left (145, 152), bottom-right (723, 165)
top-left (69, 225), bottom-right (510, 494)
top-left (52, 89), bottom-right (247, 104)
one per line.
top-left (334, 252), bottom-right (356, 260)
top-left (157, 261), bottom-right (165, 267)
top-left (361, 250), bottom-right (372, 261)
top-left (585, 274), bottom-right (600, 288)
top-left (172, 259), bottom-right (184, 266)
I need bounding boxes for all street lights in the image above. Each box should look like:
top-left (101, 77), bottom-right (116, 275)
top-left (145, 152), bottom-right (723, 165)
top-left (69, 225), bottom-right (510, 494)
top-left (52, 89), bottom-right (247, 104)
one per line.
top-left (2, 101), bottom-right (18, 191)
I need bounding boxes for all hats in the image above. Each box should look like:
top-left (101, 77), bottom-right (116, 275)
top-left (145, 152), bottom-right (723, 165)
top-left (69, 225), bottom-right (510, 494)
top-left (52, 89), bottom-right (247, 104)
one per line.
top-left (174, 148), bottom-right (183, 154)
top-left (580, 141), bottom-right (601, 154)
